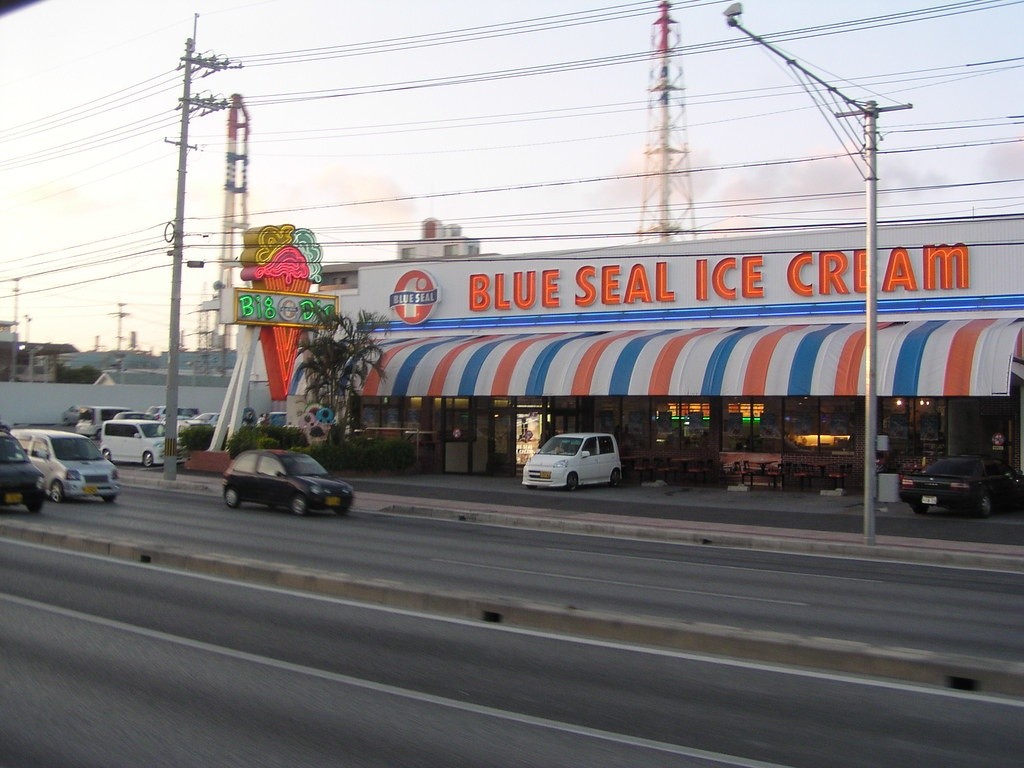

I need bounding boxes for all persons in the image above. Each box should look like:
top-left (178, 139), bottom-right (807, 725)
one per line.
top-left (256, 413), bottom-right (271, 428)
top-left (241, 410), bottom-right (257, 428)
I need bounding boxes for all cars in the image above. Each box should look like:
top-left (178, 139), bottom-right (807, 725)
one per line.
top-left (900, 454), bottom-right (1024, 518)
top-left (193, 412), bottom-right (219, 427)
top-left (521, 432), bottom-right (623, 490)
top-left (113, 412), bottom-right (157, 422)
top-left (146, 405), bottom-right (167, 421)
top-left (222, 449), bottom-right (354, 516)
top-left (0, 421), bottom-right (45, 513)
top-left (177, 407), bottom-right (199, 420)
top-left (61, 405), bottom-right (84, 426)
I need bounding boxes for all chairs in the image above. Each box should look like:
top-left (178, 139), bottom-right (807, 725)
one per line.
top-left (633, 456), bottom-right (853, 492)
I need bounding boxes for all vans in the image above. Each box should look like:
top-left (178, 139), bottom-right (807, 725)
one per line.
top-left (8, 427), bottom-right (122, 503)
top-left (99, 418), bottom-right (186, 468)
top-left (74, 407), bottom-right (130, 440)
